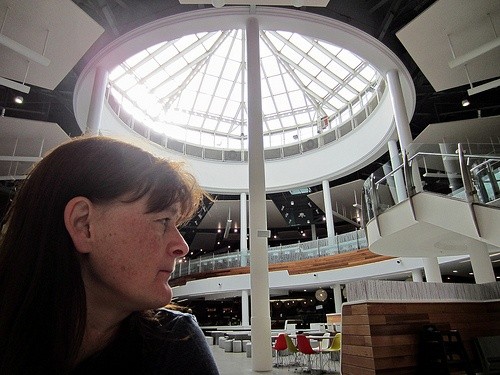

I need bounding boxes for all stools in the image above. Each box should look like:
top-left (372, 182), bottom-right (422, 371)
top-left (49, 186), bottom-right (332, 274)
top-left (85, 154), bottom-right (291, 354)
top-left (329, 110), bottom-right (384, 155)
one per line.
top-left (205, 336), bottom-right (213, 346)
top-left (219, 336), bottom-right (251, 357)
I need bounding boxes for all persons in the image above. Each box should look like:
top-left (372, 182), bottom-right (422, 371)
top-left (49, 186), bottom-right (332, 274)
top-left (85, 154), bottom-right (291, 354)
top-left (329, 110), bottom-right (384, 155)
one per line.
top-left (0, 136), bottom-right (219, 375)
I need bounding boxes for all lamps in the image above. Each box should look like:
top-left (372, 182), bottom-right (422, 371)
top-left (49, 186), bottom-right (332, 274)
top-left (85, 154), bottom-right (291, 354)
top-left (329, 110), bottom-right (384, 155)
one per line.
top-left (462, 98), bottom-right (470, 107)
top-left (15, 95), bottom-right (23, 103)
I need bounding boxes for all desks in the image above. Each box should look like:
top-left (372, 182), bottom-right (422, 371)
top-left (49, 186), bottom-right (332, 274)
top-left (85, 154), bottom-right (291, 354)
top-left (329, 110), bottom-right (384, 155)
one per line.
top-left (287, 335), bottom-right (299, 367)
top-left (206, 331), bottom-right (252, 340)
top-left (306, 336), bottom-right (334, 374)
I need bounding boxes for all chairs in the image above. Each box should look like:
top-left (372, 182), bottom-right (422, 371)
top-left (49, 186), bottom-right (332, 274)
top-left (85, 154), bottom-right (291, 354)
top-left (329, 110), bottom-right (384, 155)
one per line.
top-left (272, 333), bottom-right (342, 375)
